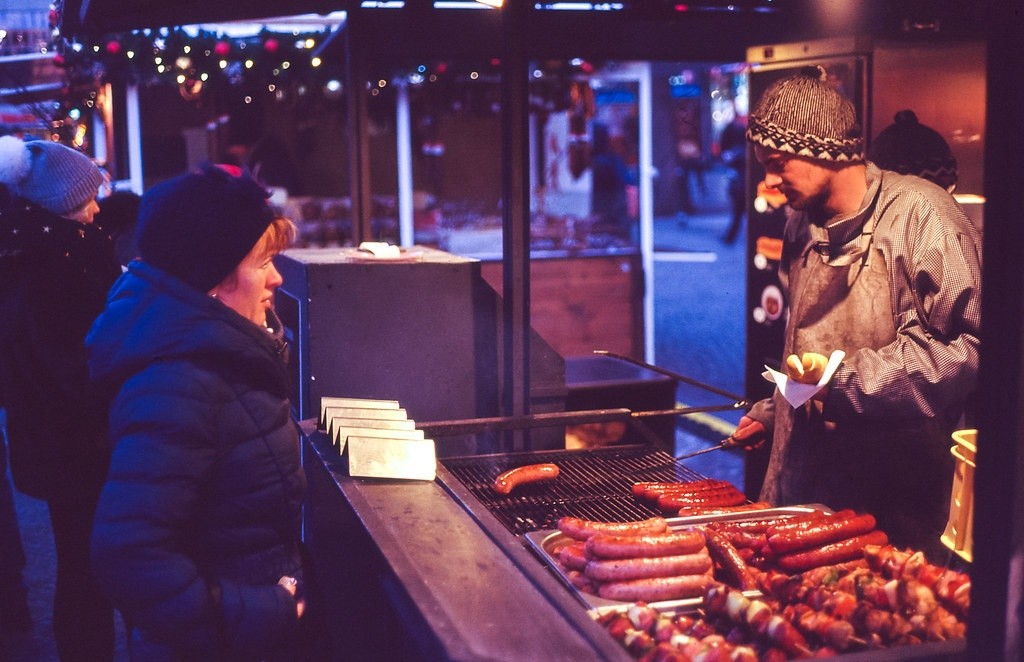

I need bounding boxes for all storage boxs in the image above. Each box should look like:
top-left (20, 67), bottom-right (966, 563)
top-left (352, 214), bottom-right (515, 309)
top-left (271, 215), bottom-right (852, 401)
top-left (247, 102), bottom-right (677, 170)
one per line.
top-left (942, 429), bottom-right (977, 565)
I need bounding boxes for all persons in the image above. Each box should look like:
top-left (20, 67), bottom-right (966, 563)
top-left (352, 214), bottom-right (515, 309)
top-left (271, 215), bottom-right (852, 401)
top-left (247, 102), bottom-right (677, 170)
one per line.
top-left (734, 66), bottom-right (982, 565)
top-left (721, 115), bottom-right (748, 243)
top-left (0, 119), bottom-right (309, 662)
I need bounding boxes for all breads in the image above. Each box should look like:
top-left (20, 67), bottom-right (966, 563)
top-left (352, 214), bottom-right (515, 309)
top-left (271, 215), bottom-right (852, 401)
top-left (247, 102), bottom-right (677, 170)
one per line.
top-left (786, 352), bottom-right (828, 384)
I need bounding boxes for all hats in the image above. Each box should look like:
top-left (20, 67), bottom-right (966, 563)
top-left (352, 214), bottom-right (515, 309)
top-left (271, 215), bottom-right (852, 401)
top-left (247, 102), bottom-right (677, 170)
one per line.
top-left (744, 63), bottom-right (867, 162)
top-left (0, 135), bottom-right (104, 215)
top-left (133, 167), bottom-right (274, 293)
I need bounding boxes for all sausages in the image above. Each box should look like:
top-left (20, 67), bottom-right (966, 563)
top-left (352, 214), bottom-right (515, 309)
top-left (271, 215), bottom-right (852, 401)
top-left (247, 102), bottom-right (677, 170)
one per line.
top-left (558, 477), bottom-right (891, 603)
top-left (494, 463), bottom-right (560, 495)
